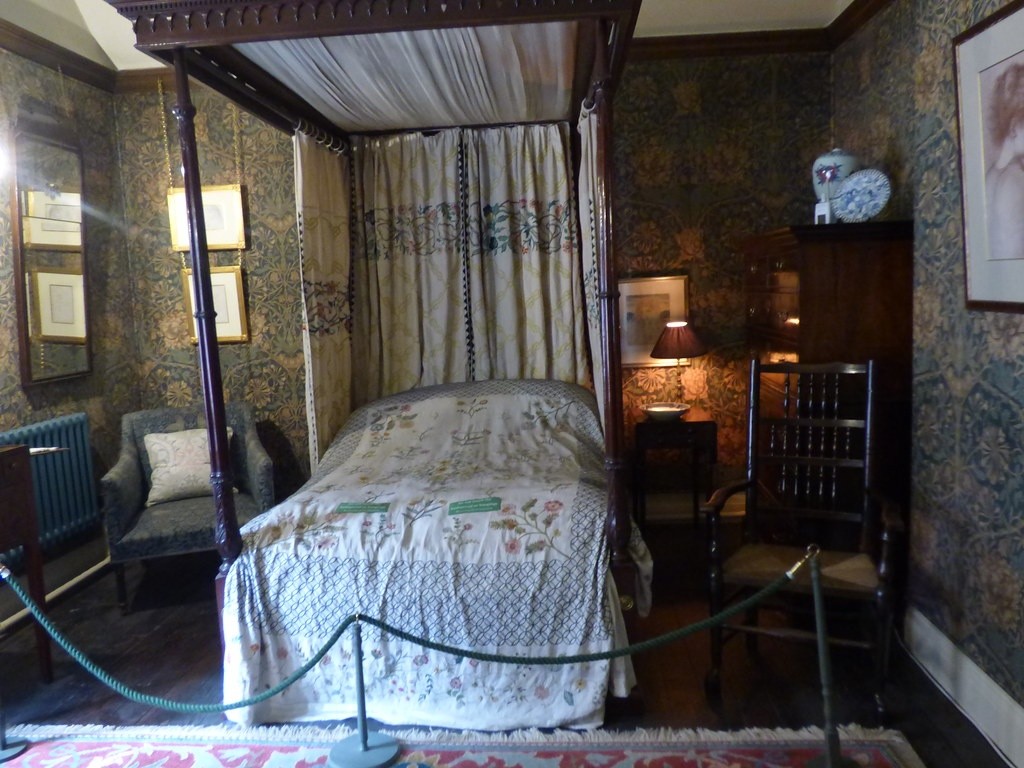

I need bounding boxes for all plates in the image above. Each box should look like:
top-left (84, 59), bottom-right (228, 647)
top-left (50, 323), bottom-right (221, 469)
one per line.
top-left (831, 169), bottom-right (890, 222)
top-left (641, 402), bottom-right (690, 419)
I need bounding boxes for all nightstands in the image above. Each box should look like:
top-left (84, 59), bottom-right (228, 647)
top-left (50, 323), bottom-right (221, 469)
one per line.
top-left (635, 420), bottom-right (719, 527)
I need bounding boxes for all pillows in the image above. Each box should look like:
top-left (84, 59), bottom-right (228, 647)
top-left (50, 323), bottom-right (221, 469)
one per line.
top-left (143, 426), bottom-right (239, 508)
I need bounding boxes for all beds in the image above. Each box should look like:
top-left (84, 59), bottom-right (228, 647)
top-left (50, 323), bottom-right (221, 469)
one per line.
top-left (220, 380), bottom-right (644, 733)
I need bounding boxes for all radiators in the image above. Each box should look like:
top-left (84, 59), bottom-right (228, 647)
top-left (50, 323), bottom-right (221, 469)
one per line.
top-left (0, 411), bottom-right (100, 567)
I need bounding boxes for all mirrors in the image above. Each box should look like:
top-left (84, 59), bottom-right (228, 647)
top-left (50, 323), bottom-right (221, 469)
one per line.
top-left (9, 118), bottom-right (93, 393)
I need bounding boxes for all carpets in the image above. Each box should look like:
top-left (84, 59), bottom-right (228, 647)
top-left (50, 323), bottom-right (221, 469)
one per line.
top-left (1, 720), bottom-right (926, 768)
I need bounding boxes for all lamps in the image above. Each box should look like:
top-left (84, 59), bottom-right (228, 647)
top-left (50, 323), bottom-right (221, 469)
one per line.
top-left (651, 321), bottom-right (708, 421)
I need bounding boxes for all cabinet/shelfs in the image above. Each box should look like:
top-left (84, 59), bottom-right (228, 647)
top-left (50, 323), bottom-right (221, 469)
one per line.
top-left (739, 217), bottom-right (914, 627)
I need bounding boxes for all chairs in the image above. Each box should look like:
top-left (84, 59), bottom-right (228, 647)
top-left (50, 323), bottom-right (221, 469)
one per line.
top-left (100, 400), bottom-right (276, 616)
top-left (699, 356), bottom-right (904, 732)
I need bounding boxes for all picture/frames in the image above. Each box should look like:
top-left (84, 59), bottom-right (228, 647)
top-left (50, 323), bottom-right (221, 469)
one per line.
top-left (947, 0), bottom-right (1024, 315)
top-left (19, 186), bottom-right (83, 253)
top-left (31, 266), bottom-right (88, 345)
top-left (616, 274), bottom-right (691, 368)
top-left (167, 183), bottom-right (246, 252)
top-left (180, 266), bottom-right (250, 344)
